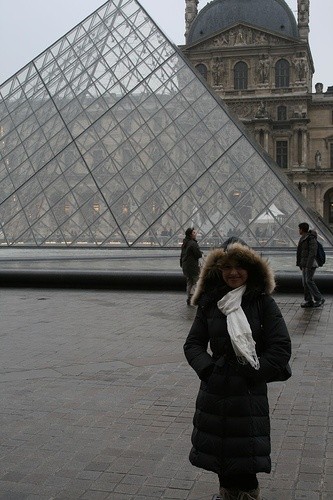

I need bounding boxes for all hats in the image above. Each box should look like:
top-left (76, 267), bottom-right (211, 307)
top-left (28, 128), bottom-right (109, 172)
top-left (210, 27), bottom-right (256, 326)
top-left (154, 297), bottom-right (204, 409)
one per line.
top-left (185, 228), bottom-right (194, 234)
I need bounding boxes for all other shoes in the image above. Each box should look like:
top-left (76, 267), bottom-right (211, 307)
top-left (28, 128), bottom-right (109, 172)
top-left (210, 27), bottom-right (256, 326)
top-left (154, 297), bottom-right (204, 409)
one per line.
top-left (313, 299), bottom-right (325, 307)
top-left (301, 301), bottom-right (313, 308)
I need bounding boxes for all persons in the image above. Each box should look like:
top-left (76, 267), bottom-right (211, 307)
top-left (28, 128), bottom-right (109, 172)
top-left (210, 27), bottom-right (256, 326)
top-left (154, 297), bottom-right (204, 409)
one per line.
top-left (214, 25), bottom-right (268, 45)
top-left (147, 226), bottom-right (270, 244)
top-left (296, 223), bottom-right (326, 308)
top-left (180, 228), bottom-right (205, 305)
top-left (183, 236), bottom-right (292, 500)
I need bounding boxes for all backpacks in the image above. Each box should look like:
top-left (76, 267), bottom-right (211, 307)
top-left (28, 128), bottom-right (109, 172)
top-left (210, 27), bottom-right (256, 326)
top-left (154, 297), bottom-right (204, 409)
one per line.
top-left (307, 237), bottom-right (325, 266)
